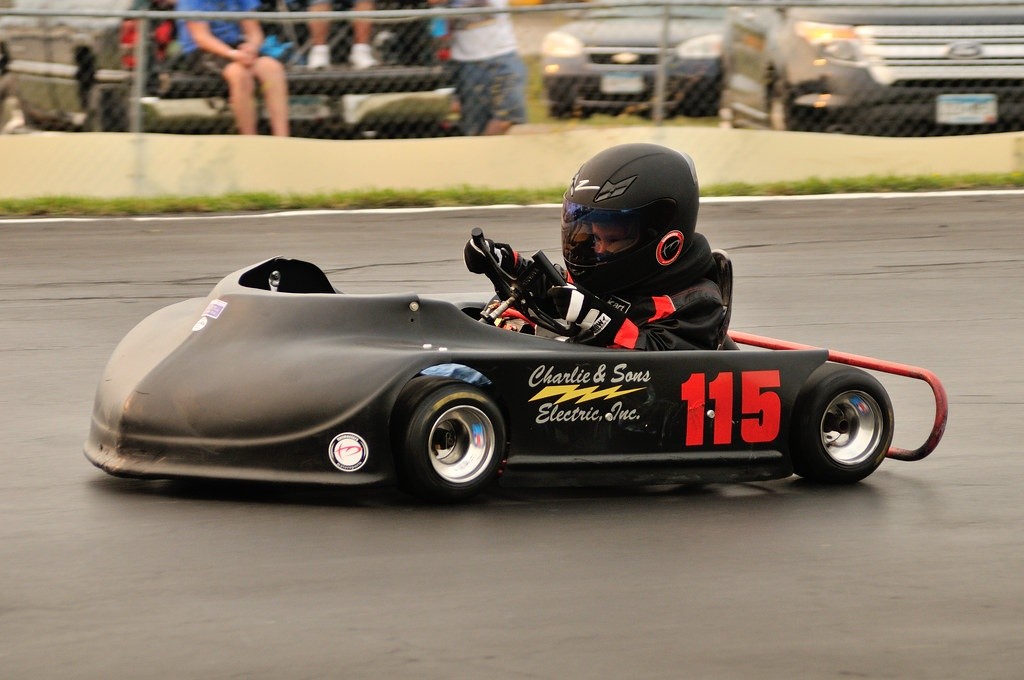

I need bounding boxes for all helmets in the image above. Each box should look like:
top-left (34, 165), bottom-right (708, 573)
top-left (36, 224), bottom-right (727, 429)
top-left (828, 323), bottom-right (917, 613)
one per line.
top-left (562, 142), bottom-right (699, 292)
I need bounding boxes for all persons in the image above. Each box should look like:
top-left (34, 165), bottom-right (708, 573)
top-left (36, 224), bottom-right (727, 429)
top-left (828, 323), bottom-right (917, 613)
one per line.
top-left (173, 0), bottom-right (526, 138)
top-left (463, 142), bottom-right (726, 350)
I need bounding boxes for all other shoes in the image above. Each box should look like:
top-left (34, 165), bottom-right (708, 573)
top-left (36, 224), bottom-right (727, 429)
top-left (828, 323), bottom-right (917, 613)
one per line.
top-left (304, 45), bottom-right (331, 71)
top-left (261, 36), bottom-right (297, 62)
top-left (348, 44), bottom-right (379, 71)
top-left (372, 31), bottom-right (396, 55)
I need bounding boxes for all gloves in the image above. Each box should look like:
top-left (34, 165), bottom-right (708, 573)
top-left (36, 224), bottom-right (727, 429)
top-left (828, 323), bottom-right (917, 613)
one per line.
top-left (461, 234), bottom-right (517, 287)
top-left (544, 280), bottom-right (625, 344)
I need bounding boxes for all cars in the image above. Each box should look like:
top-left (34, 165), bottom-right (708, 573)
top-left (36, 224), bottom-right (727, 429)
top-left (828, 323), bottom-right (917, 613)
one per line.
top-left (540, 1), bottom-right (726, 119)
top-left (0, 0), bottom-right (465, 137)
top-left (720, 1), bottom-right (1024, 137)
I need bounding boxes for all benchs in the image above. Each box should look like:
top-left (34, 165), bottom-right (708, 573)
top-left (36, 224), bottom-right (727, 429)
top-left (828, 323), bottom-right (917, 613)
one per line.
top-left (151, 63), bottom-right (446, 97)
